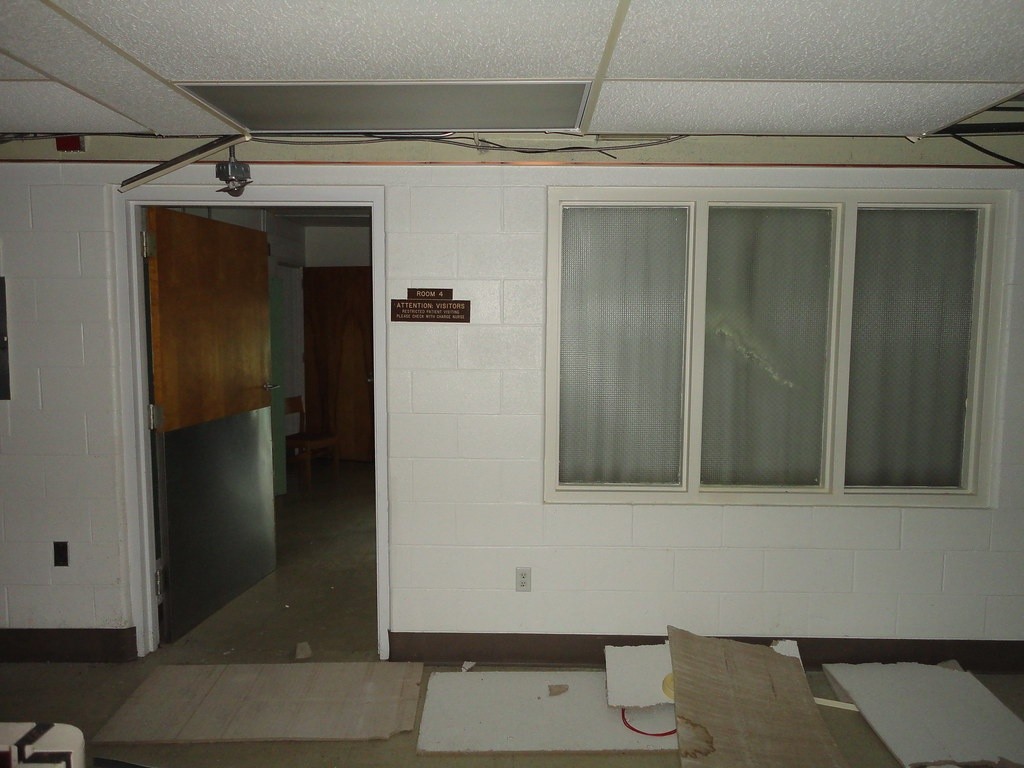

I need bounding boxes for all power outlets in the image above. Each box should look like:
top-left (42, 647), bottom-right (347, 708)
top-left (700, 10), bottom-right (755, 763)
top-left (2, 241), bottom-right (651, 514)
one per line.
top-left (516, 567), bottom-right (531, 592)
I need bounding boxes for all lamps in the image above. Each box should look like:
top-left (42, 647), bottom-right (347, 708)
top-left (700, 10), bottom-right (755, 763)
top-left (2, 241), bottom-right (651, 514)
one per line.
top-left (215, 145), bottom-right (253, 197)
top-left (117, 132), bottom-right (252, 195)
